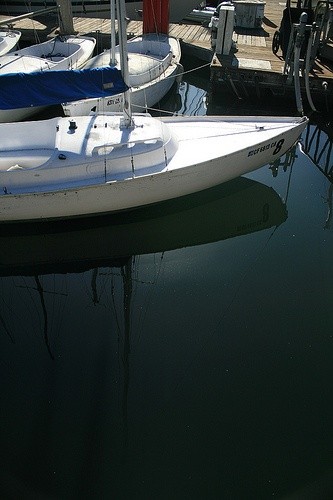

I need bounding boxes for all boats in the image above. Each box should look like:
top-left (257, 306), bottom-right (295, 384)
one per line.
top-left (3, 1), bottom-right (203, 23)
top-left (2, 177), bottom-right (291, 471)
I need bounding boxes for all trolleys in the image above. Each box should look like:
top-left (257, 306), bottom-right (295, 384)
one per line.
top-left (272, 1), bottom-right (317, 59)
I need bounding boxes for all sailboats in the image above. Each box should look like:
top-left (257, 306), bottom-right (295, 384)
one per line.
top-left (0, 0), bottom-right (308, 222)
top-left (1, 34), bottom-right (96, 123)
top-left (60, 0), bottom-right (184, 116)
top-left (1, 27), bottom-right (22, 57)
